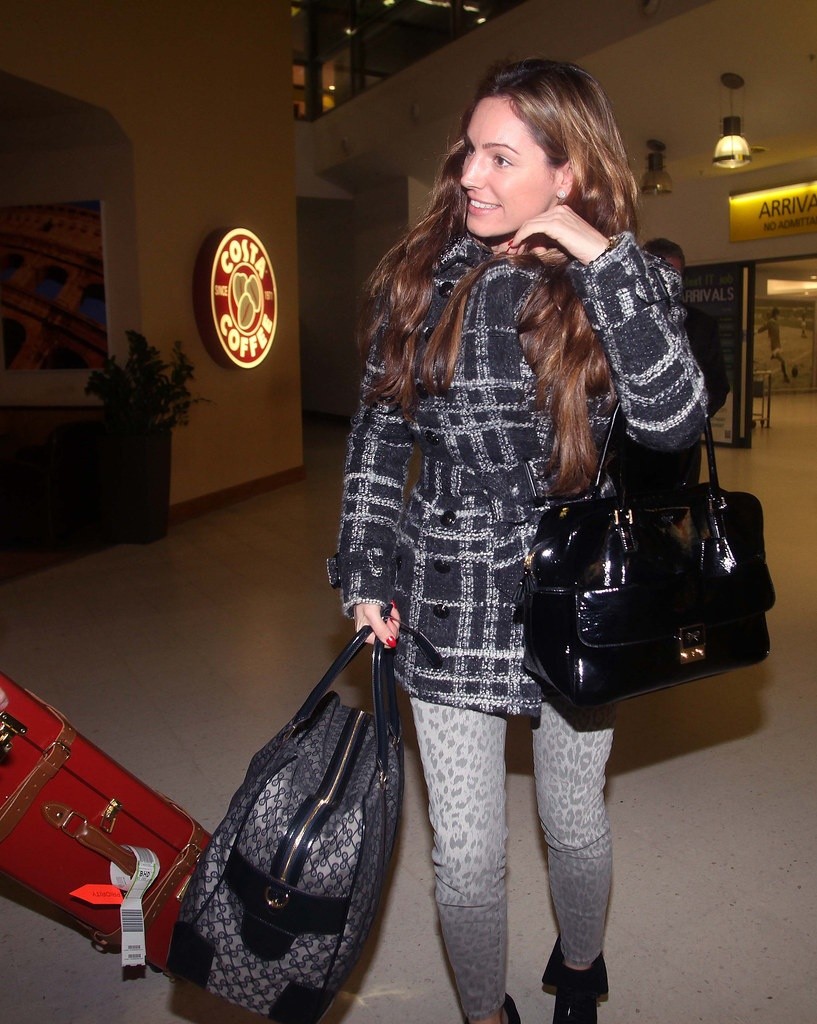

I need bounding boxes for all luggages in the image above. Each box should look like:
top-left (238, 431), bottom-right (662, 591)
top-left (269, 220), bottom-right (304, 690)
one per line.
top-left (0, 673), bottom-right (215, 984)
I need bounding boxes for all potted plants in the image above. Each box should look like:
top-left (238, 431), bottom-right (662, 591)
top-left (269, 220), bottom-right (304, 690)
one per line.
top-left (85, 332), bottom-right (212, 546)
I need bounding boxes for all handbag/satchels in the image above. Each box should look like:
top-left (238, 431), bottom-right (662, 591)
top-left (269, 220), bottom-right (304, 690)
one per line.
top-left (165, 604), bottom-right (405, 1024)
top-left (511, 398), bottom-right (777, 709)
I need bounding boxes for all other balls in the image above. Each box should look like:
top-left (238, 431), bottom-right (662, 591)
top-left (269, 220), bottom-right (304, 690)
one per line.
top-left (791, 366), bottom-right (798, 376)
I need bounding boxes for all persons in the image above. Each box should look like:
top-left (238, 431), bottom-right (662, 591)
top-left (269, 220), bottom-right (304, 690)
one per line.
top-left (757, 308), bottom-right (790, 384)
top-left (611, 239), bottom-right (730, 496)
top-left (327, 61), bottom-right (708, 1024)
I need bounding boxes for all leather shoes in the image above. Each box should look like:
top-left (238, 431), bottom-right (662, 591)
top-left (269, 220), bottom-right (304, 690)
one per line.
top-left (465, 992), bottom-right (521, 1024)
top-left (542, 934), bottom-right (608, 1024)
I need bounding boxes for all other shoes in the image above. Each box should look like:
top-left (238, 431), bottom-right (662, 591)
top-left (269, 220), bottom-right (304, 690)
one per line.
top-left (771, 354), bottom-right (774, 360)
top-left (784, 377), bottom-right (790, 383)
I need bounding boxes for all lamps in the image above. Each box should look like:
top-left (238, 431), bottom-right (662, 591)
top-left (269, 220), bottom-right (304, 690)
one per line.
top-left (638, 138), bottom-right (671, 194)
top-left (712, 72), bottom-right (752, 168)
